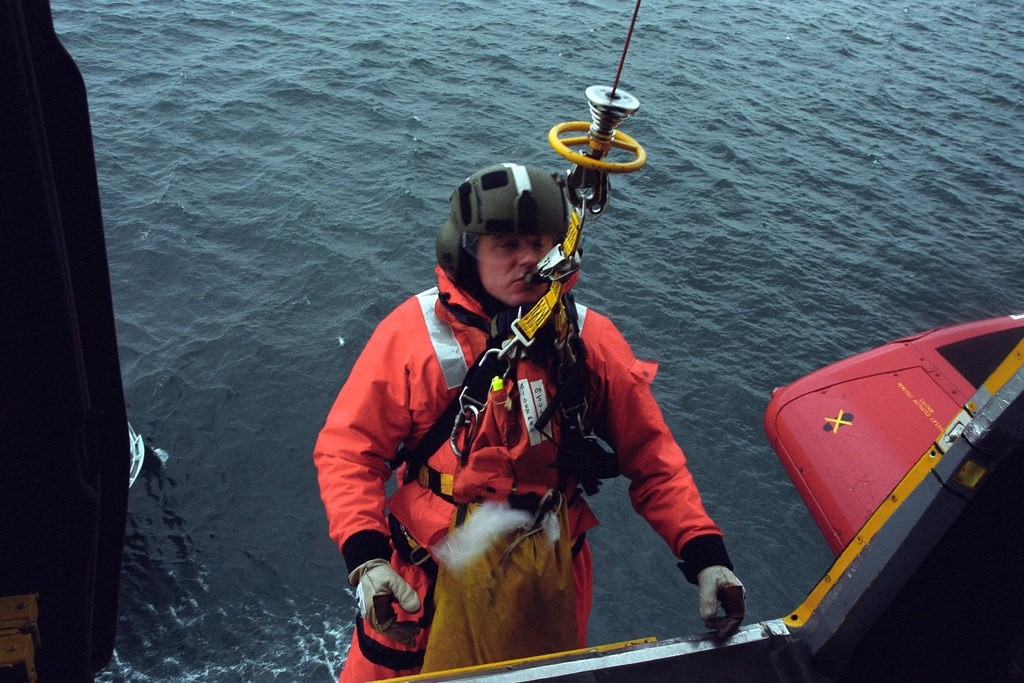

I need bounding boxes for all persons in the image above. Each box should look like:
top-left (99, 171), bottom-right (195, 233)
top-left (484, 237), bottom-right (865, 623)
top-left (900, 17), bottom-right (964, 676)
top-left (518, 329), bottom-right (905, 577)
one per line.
top-left (315, 163), bottom-right (747, 682)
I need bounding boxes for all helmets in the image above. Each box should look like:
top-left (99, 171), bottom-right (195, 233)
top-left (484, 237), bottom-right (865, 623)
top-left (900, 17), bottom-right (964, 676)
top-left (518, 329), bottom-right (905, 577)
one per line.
top-left (435, 162), bottom-right (569, 284)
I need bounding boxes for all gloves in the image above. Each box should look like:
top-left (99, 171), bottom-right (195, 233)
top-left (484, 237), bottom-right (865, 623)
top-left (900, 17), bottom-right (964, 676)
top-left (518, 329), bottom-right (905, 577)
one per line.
top-left (696, 566), bottom-right (746, 644)
top-left (347, 559), bottom-right (421, 649)
